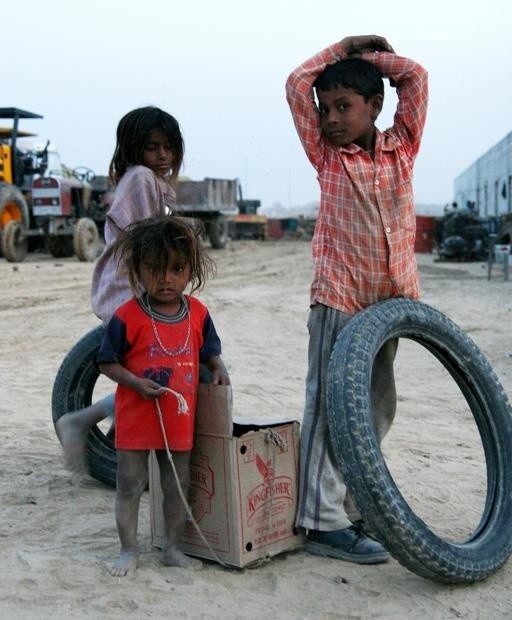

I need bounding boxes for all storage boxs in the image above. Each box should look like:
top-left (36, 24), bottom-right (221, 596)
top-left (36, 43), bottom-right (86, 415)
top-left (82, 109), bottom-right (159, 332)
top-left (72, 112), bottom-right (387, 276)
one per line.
top-left (148, 384), bottom-right (307, 569)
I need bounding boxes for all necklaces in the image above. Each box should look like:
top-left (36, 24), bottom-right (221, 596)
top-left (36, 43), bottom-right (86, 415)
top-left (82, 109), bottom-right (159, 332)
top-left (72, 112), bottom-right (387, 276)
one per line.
top-left (141, 291), bottom-right (194, 357)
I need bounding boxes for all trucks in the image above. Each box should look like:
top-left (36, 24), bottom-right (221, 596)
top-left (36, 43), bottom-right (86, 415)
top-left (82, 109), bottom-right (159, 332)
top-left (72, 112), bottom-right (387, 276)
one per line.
top-left (79, 168), bottom-right (237, 248)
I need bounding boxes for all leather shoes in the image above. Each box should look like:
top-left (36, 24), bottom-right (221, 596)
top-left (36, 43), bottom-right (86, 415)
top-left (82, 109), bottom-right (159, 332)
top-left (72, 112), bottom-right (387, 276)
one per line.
top-left (304, 524), bottom-right (387, 563)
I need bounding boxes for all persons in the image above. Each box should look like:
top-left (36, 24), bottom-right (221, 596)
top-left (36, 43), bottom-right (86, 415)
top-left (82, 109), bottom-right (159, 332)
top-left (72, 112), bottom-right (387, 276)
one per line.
top-left (295, 215), bottom-right (306, 240)
top-left (283, 32), bottom-right (430, 568)
top-left (463, 200), bottom-right (481, 216)
top-left (54, 106), bottom-right (186, 486)
top-left (94, 213), bottom-right (232, 579)
top-left (443, 200), bottom-right (460, 216)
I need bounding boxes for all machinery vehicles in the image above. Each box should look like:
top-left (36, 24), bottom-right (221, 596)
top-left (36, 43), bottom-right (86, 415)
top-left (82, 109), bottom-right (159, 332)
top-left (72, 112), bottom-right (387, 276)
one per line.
top-left (1, 107), bottom-right (99, 261)
top-left (229, 178), bottom-right (268, 240)
